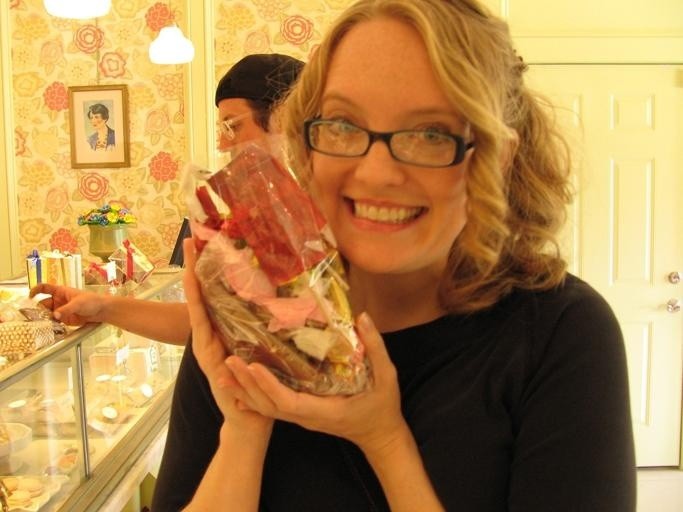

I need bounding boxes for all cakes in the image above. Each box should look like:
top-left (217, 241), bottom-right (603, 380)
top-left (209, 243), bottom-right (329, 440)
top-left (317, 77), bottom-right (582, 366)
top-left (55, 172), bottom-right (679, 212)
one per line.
top-left (0, 476), bottom-right (42, 510)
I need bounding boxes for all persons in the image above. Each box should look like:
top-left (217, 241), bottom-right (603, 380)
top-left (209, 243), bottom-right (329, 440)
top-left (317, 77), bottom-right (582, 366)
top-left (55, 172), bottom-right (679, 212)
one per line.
top-left (151, 0), bottom-right (637, 512)
top-left (29, 54), bottom-right (307, 348)
top-left (87, 104), bottom-right (115, 152)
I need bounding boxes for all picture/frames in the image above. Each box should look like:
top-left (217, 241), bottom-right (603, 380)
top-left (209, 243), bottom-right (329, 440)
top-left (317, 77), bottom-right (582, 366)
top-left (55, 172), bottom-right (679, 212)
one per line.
top-left (67, 83), bottom-right (131, 168)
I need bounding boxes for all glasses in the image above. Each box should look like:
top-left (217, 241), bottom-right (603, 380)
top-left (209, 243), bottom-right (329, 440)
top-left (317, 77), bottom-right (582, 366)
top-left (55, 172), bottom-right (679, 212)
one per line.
top-left (215, 109), bottom-right (264, 146)
top-left (303, 114), bottom-right (474, 168)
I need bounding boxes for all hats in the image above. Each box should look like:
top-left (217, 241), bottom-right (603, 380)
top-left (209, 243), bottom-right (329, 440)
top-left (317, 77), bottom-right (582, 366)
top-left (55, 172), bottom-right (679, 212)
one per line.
top-left (216, 54), bottom-right (306, 104)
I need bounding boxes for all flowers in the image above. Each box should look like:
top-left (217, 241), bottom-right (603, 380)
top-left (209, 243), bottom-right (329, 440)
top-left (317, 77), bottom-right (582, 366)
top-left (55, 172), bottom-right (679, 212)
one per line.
top-left (78, 202), bottom-right (137, 227)
